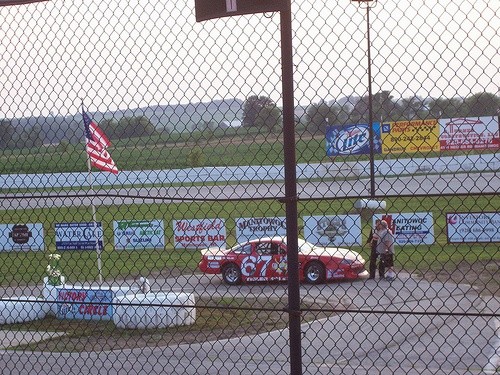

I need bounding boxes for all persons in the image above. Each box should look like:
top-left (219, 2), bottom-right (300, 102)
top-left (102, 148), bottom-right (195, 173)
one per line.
top-left (367, 219), bottom-right (395, 281)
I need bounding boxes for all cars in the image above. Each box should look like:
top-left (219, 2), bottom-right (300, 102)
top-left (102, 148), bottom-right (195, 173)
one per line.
top-left (199, 236), bottom-right (365, 286)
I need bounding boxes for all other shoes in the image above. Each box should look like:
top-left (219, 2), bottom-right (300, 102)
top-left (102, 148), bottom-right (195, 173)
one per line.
top-left (385, 275), bottom-right (395, 281)
top-left (368, 277), bottom-right (374, 279)
top-left (378, 276), bottom-right (384, 279)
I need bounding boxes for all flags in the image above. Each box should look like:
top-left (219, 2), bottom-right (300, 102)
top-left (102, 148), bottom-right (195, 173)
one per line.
top-left (83, 110), bottom-right (118, 174)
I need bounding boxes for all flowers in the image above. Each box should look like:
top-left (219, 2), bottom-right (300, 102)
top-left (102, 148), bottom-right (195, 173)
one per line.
top-left (47, 254), bottom-right (65, 285)
